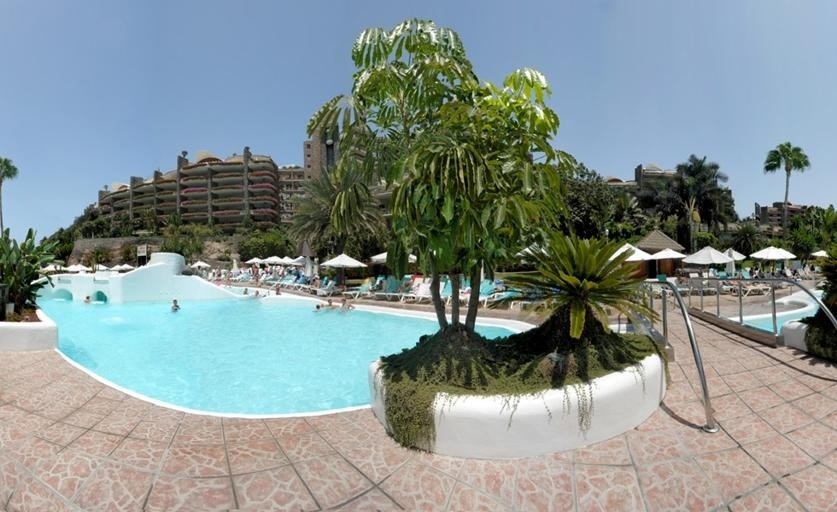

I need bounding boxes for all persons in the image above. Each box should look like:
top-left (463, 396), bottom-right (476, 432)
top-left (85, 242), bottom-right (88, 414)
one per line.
top-left (190, 263), bottom-right (415, 313)
top-left (83, 295), bottom-right (91, 305)
top-left (171, 298), bottom-right (180, 313)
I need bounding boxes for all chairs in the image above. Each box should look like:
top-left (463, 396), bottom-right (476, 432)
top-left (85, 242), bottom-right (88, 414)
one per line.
top-left (211, 267), bottom-right (342, 297)
top-left (343, 273), bottom-right (529, 310)
top-left (644, 266), bottom-right (823, 297)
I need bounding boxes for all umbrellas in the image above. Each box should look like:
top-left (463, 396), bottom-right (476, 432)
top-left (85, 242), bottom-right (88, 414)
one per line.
top-left (190, 249), bottom-right (417, 285)
top-left (38, 262), bottom-right (134, 274)
